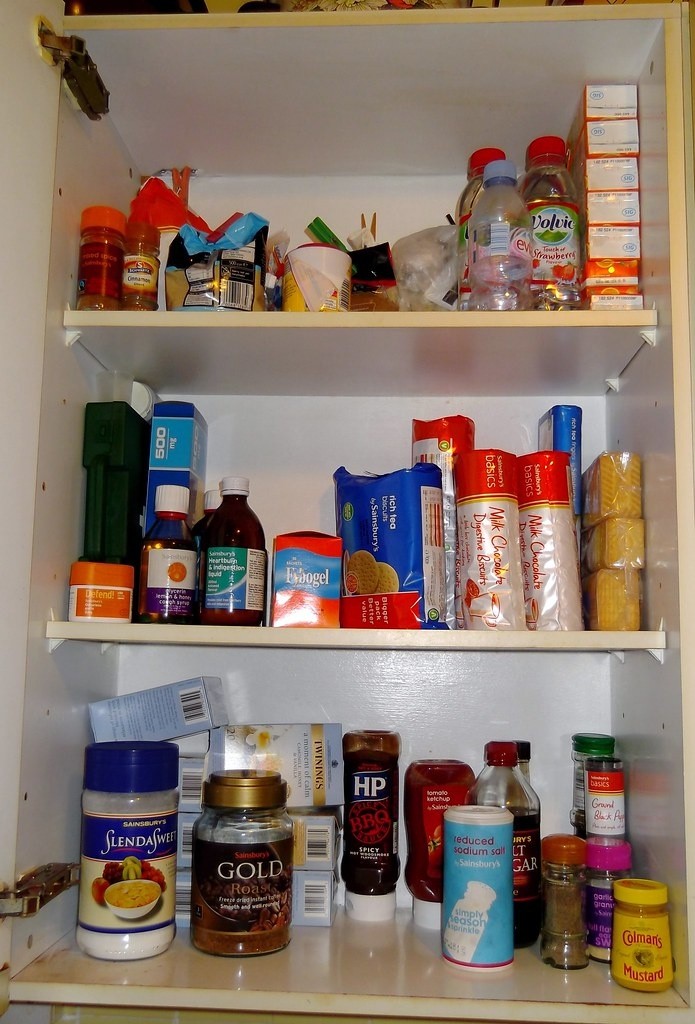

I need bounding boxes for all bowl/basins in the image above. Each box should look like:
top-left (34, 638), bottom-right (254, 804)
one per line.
top-left (103, 880), bottom-right (160, 922)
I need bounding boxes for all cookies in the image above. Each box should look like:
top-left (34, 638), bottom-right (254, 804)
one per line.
top-left (348, 551), bottom-right (399, 596)
top-left (578, 450), bottom-right (645, 632)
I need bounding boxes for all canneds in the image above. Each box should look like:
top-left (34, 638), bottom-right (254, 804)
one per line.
top-left (77, 741), bottom-right (179, 960)
top-left (585, 838), bottom-right (674, 992)
top-left (74, 206), bottom-right (160, 310)
top-left (189, 768), bottom-right (294, 957)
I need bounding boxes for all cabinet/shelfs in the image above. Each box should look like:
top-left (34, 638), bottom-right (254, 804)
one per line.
top-left (0, 0), bottom-right (695, 1024)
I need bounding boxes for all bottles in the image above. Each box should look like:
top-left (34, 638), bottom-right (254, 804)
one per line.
top-left (121, 225), bottom-right (160, 309)
top-left (518, 137), bottom-right (583, 311)
top-left (140, 484), bottom-right (201, 622)
top-left (541, 834), bottom-right (590, 969)
top-left (76, 205), bottom-right (126, 310)
top-left (193, 487), bottom-right (220, 621)
top-left (465, 740), bottom-right (542, 949)
top-left (570, 733), bottom-right (615, 813)
top-left (586, 837), bottom-right (632, 960)
top-left (455, 147), bottom-right (506, 310)
top-left (200, 478), bottom-right (267, 623)
top-left (584, 756), bottom-right (624, 837)
top-left (469, 161), bottom-right (532, 309)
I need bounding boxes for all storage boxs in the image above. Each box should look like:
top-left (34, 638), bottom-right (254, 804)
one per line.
top-left (177, 808), bottom-right (341, 870)
top-left (175, 870), bottom-right (339, 926)
top-left (144, 401), bottom-right (208, 533)
top-left (209, 723), bottom-right (345, 809)
top-left (270, 531), bottom-right (344, 628)
top-left (89, 677), bottom-right (229, 744)
